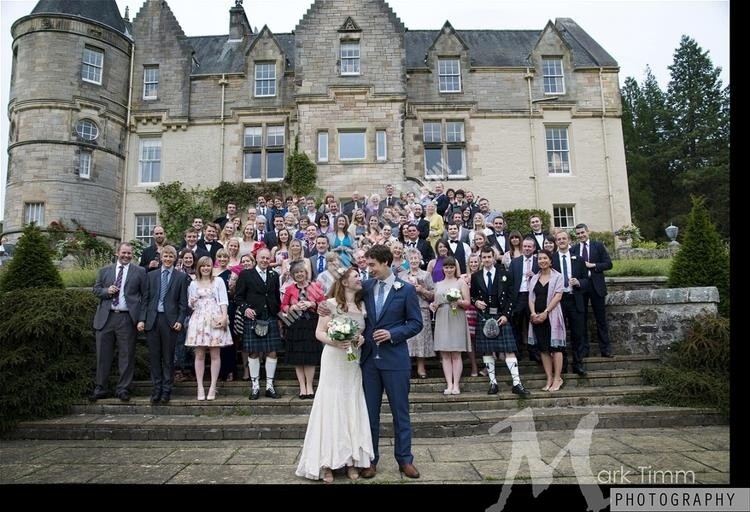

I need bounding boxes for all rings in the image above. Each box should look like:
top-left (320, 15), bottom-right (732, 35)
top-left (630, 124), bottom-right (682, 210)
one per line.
top-left (110, 286), bottom-right (112, 289)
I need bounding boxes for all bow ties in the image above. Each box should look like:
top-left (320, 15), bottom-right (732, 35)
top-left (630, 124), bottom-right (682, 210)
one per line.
top-left (535, 233), bottom-right (542, 235)
top-left (497, 233), bottom-right (503, 236)
top-left (451, 240), bottom-right (459, 243)
top-left (205, 242), bottom-right (214, 245)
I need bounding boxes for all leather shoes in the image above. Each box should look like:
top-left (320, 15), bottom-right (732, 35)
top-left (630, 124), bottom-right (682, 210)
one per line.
top-left (151, 391), bottom-right (161, 402)
top-left (488, 383), bottom-right (498, 394)
top-left (529, 351), bottom-right (541, 361)
top-left (512, 383), bottom-right (530, 395)
top-left (443, 389), bottom-right (451, 395)
top-left (471, 373), bottom-right (478, 377)
top-left (265, 388), bottom-right (281, 399)
top-left (399, 462), bottom-right (419, 478)
top-left (88, 393), bottom-right (111, 400)
top-left (118, 391), bottom-right (130, 401)
top-left (360, 463), bottom-right (376, 478)
top-left (561, 367), bottom-right (568, 373)
top-left (300, 393), bottom-right (307, 399)
top-left (160, 391), bottom-right (171, 402)
top-left (601, 350), bottom-right (614, 358)
top-left (249, 388), bottom-right (260, 400)
top-left (573, 362), bottom-right (587, 376)
top-left (306, 392), bottom-right (315, 399)
top-left (420, 374), bottom-right (427, 378)
top-left (479, 371), bottom-right (486, 377)
top-left (452, 389), bottom-right (461, 394)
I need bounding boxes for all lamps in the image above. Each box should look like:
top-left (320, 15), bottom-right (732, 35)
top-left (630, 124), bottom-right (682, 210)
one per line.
top-left (665, 221), bottom-right (678, 240)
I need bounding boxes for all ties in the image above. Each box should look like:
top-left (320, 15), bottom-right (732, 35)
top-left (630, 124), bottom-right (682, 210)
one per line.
top-left (582, 242), bottom-right (588, 262)
top-left (526, 258), bottom-right (530, 289)
top-left (562, 255), bottom-right (569, 287)
top-left (375, 282), bottom-right (386, 322)
top-left (261, 271), bottom-right (266, 283)
top-left (263, 209), bottom-right (264, 215)
top-left (487, 271), bottom-right (493, 294)
top-left (160, 269), bottom-right (170, 303)
top-left (112, 266), bottom-right (123, 306)
top-left (319, 255), bottom-right (324, 273)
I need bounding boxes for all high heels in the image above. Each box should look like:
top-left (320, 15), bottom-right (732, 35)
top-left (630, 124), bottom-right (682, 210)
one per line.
top-left (197, 388), bottom-right (206, 401)
top-left (541, 382), bottom-right (553, 391)
top-left (226, 372), bottom-right (234, 381)
top-left (548, 377), bottom-right (564, 391)
top-left (323, 467), bottom-right (333, 482)
top-left (347, 465), bottom-right (359, 479)
top-left (206, 388), bottom-right (216, 400)
top-left (241, 376), bottom-right (250, 381)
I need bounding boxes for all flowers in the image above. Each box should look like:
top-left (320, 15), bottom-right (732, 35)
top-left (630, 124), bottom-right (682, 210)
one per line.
top-left (328, 317), bottom-right (358, 361)
top-left (391, 280), bottom-right (404, 291)
top-left (441, 288), bottom-right (463, 315)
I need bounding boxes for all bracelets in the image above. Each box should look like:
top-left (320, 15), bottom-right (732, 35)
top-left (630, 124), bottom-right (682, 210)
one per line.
top-left (544, 309), bottom-right (549, 313)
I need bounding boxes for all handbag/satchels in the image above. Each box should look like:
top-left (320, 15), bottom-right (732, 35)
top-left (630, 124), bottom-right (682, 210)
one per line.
top-left (483, 317), bottom-right (501, 339)
top-left (251, 319), bottom-right (270, 337)
top-left (211, 314), bottom-right (231, 328)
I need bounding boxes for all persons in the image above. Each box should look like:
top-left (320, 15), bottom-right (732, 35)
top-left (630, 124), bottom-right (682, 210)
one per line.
top-left (527, 250), bottom-right (572, 391)
top-left (468, 246), bottom-right (531, 396)
top-left (297, 267), bottom-right (370, 484)
top-left (135, 244), bottom-right (191, 404)
top-left (104, 180), bottom-right (615, 400)
top-left (428, 254), bottom-right (472, 397)
top-left (185, 255), bottom-right (234, 402)
top-left (311, 242), bottom-right (426, 481)
top-left (230, 247), bottom-right (284, 403)
top-left (89, 241), bottom-right (146, 405)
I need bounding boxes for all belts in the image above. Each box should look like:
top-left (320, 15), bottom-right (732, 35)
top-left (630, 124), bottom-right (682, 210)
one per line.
top-left (110, 310), bottom-right (128, 313)
